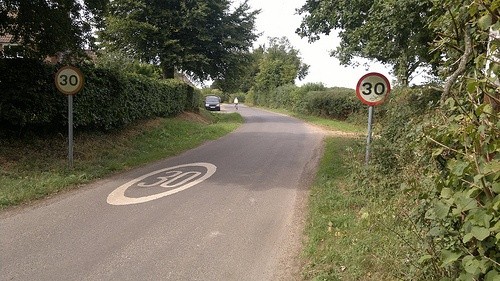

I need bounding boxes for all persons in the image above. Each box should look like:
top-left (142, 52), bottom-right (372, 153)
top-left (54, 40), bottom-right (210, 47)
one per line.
top-left (233, 96), bottom-right (238, 110)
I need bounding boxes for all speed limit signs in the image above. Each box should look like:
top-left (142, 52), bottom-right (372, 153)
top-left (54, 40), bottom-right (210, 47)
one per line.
top-left (356, 71), bottom-right (390, 107)
top-left (55, 65), bottom-right (83, 95)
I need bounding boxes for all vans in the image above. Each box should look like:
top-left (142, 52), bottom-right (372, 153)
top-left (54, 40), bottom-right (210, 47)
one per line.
top-left (203, 95), bottom-right (221, 111)
